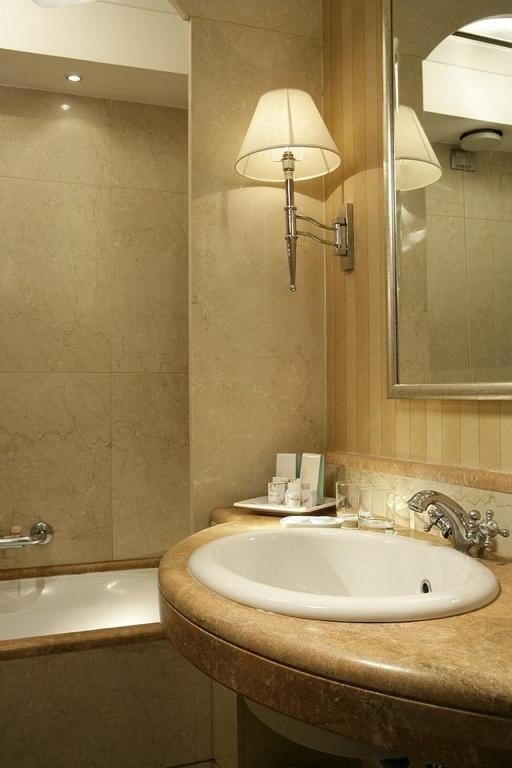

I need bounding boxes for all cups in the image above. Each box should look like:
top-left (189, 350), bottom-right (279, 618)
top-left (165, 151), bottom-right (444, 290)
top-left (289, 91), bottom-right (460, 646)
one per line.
top-left (336, 480), bottom-right (396, 532)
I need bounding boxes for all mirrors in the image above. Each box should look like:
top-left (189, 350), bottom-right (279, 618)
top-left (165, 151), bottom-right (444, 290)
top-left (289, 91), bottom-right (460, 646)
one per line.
top-left (381, 3), bottom-right (512, 404)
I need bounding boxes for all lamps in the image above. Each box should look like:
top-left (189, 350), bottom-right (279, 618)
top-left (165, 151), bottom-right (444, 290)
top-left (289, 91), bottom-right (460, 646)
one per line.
top-left (458, 128), bottom-right (503, 153)
top-left (233, 87), bottom-right (354, 289)
top-left (394, 103), bottom-right (441, 240)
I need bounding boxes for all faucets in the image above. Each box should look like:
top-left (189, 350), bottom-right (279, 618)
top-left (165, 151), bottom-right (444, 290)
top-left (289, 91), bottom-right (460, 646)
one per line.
top-left (405, 491), bottom-right (509, 561)
top-left (0, 520), bottom-right (53, 548)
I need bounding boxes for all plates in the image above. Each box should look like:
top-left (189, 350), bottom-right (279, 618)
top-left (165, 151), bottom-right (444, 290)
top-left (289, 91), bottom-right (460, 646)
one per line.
top-left (281, 516), bottom-right (344, 527)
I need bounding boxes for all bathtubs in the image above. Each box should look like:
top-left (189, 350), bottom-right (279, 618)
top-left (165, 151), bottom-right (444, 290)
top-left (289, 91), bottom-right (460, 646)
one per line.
top-left (1, 558), bottom-right (166, 646)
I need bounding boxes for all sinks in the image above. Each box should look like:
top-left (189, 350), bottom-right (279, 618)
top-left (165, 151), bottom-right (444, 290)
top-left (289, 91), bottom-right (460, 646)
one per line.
top-left (188, 525), bottom-right (498, 623)
